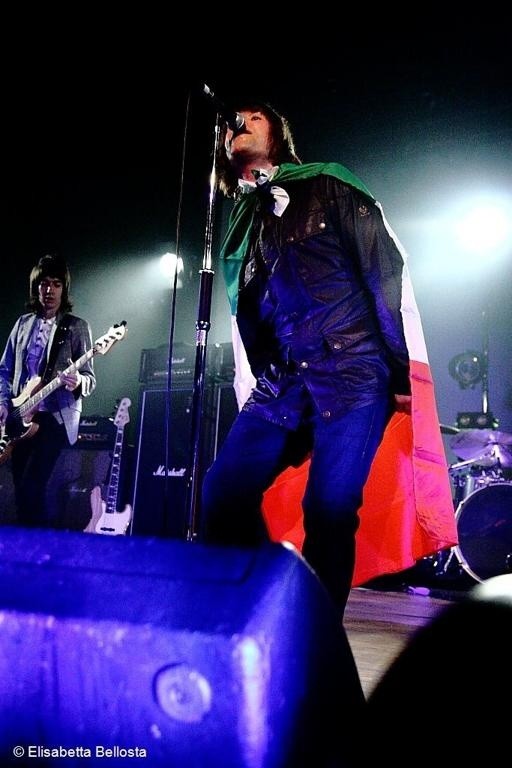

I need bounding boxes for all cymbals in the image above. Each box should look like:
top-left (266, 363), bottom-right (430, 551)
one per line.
top-left (440, 424), bottom-right (460, 436)
top-left (449, 429), bottom-right (511, 468)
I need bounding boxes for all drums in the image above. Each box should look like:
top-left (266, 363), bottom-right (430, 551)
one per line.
top-left (451, 481), bottom-right (512, 584)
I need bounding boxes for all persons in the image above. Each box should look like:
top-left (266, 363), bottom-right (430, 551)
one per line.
top-left (0, 255), bottom-right (96, 524)
top-left (201, 102), bottom-right (409, 621)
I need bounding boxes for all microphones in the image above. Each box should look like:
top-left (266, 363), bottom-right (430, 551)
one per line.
top-left (198, 82), bottom-right (245, 131)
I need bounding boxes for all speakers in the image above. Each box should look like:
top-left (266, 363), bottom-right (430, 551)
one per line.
top-left (332, 573), bottom-right (512, 768)
top-left (0, 525), bottom-right (368, 768)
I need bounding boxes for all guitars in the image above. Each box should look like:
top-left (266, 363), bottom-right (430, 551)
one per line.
top-left (84, 397), bottom-right (132, 537)
top-left (0, 321), bottom-right (127, 459)
